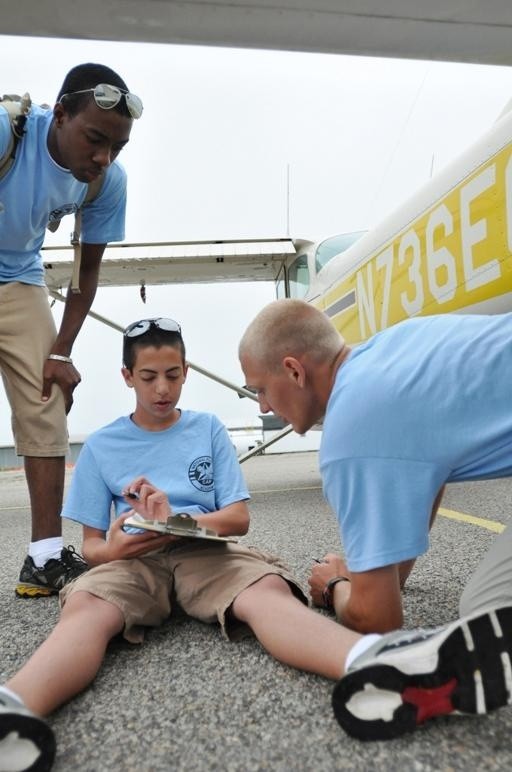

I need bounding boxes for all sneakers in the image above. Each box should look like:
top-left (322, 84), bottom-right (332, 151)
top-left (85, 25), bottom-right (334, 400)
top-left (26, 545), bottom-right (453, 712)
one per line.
top-left (15, 545), bottom-right (90, 599)
top-left (330, 602), bottom-right (511, 742)
top-left (0, 687), bottom-right (56, 772)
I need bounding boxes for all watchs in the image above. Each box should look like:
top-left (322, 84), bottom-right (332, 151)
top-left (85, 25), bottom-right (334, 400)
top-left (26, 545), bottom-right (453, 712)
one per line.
top-left (321, 577), bottom-right (350, 615)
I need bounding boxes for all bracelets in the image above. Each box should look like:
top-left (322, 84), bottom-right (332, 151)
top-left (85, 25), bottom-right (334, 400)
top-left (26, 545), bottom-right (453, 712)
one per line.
top-left (46, 353), bottom-right (72, 363)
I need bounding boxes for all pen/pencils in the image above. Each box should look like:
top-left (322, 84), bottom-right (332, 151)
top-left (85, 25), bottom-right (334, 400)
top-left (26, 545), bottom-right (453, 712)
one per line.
top-left (312, 556), bottom-right (328, 564)
top-left (122, 491), bottom-right (138, 499)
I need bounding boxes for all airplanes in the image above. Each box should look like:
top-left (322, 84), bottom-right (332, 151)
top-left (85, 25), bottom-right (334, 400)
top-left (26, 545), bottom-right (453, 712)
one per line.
top-left (1, 0), bottom-right (512, 488)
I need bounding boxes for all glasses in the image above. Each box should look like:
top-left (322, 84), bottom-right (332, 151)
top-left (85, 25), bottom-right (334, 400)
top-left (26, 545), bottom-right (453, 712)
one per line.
top-left (122, 317), bottom-right (182, 366)
top-left (58, 82), bottom-right (144, 120)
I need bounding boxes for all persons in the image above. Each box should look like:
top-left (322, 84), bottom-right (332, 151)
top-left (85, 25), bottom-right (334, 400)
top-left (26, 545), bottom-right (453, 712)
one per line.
top-left (239, 300), bottom-right (512, 636)
top-left (0, 316), bottom-right (512, 771)
top-left (0, 63), bottom-right (143, 599)
